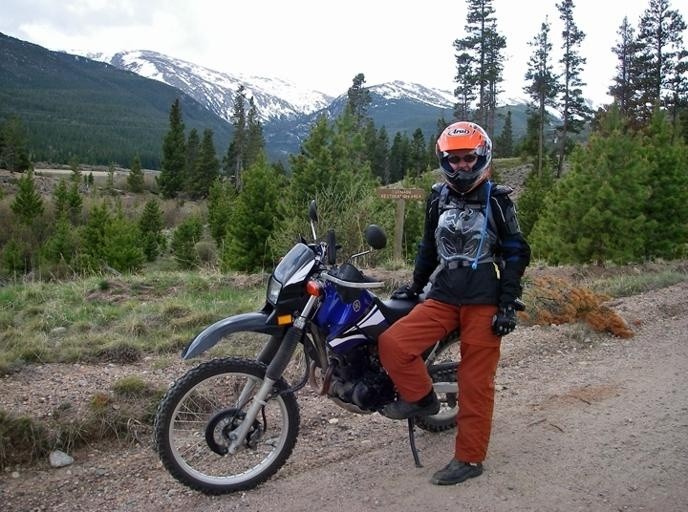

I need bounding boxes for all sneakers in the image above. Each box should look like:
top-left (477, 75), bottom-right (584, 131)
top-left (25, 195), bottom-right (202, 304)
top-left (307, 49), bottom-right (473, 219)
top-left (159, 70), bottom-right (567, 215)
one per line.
top-left (432, 457), bottom-right (484, 485)
top-left (383, 385), bottom-right (440, 419)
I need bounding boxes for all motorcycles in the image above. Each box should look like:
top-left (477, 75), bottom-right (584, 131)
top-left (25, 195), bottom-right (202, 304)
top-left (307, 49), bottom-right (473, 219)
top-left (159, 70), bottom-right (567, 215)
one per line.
top-left (153, 200), bottom-right (525, 495)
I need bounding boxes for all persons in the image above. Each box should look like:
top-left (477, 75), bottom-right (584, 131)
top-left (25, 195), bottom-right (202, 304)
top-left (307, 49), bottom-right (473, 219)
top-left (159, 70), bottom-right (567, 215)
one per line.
top-left (375, 121), bottom-right (532, 485)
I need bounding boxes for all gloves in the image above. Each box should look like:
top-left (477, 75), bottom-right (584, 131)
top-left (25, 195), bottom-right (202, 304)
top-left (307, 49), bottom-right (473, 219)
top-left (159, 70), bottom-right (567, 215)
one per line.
top-left (491, 304), bottom-right (517, 337)
top-left (390, 282), bottom-right (419, 300)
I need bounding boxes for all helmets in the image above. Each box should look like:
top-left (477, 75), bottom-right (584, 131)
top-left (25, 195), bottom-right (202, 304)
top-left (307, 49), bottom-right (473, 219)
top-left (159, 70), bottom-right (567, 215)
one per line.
top-left (435, 121), bottom-right (493, 195)
